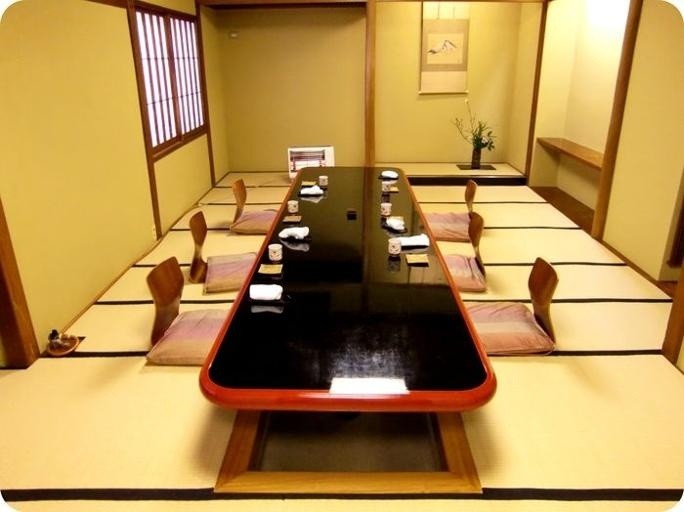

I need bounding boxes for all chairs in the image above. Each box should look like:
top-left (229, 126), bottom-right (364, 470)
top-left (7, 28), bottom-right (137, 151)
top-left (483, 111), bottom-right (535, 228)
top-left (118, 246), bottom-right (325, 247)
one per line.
top-left (228, 177), bottom-right (277, 233)
top-left (186, 209), bottom-right (256, 292)
top-left (466, 258), bottom-right (558, 357)
top-left (441, 213), bottom-right (489, 294)
top-left (147, 257), bottom-right (226, 368)
top-left (423, 179), bottom-right (478, 244)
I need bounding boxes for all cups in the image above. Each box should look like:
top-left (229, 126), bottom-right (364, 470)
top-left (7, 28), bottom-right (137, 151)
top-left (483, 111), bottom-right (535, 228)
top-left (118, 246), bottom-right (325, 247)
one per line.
top-left (388, 237), bottom-right (402, 258)
top-left (380, 201), bottom-right (393, 217)
top-left (381, 180), bottom-right (391, 195)
top-left (268, 243), bottom-right (283, 262)
top-left (287, 200), bottom-right (300, 214)
top-left (318, 175), bottom-right (329, 188)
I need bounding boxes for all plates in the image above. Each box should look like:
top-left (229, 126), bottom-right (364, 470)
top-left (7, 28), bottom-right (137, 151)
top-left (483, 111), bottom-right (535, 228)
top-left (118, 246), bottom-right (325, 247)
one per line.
top-left (283, 215), bottom-right (303, 223)
top-left (404, 252), bottom-right (429, 266)
top-left (257, 263), bottom-right (284, 275)
top-left (301, 180), bottom-right (316, 187)
top-left (381, 185), bottom-right (399, 193)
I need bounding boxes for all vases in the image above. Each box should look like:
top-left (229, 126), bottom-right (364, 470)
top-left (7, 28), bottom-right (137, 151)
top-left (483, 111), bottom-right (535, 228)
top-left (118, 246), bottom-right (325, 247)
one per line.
top-left (470, 149), bottom-right (483, 169)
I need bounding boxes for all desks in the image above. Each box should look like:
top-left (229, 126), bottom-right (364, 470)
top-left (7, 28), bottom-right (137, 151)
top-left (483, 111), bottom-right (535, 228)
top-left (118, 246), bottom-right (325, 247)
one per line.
top-left (200, 168), bottom-right (498, 410)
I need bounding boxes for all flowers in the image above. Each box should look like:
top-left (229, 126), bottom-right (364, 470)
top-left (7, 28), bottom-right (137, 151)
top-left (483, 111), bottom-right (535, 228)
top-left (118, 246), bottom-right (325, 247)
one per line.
top-left (451, 98), bottom-right (497, 149)
